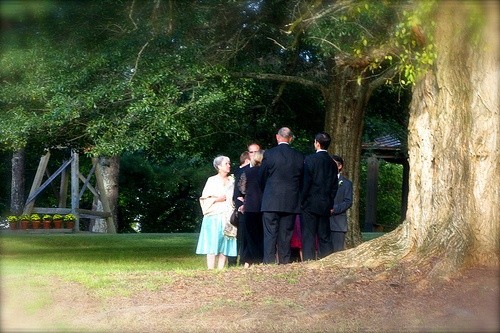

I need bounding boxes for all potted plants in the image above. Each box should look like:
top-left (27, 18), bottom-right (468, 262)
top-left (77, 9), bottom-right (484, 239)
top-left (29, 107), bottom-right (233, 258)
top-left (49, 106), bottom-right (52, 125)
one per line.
top-left (64, 214), bottom-right (77, 229)
top-left (19, 214), bottom-right (30, 229)
top-left (52, 214), bottom-right (63, 228)
top-left (6, 216), bottom-right (18, 230)
top-left (30, 213), bottom-right (41, 228)
top-left (42, 214), bottom-right (52, 229)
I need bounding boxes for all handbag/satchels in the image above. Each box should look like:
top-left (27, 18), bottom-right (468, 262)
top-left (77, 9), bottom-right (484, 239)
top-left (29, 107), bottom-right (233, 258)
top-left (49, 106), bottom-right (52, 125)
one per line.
top-left (230, 209), bottom-right (238, 227)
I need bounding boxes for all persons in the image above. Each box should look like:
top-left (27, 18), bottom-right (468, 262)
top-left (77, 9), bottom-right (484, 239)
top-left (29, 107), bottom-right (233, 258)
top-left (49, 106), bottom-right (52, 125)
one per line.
top-left (257, 127), bottom-right (304, 262)
top-left (196, 156), bottom-right (238, 269)
top-left (329, 155), bottom-right (352, 253)
top-left (277, 215), bottom-right (318, 263)
top-left (227, 144), bottom-right (267, 268)
top-left (299, 132), bottom-right (338, 261)
